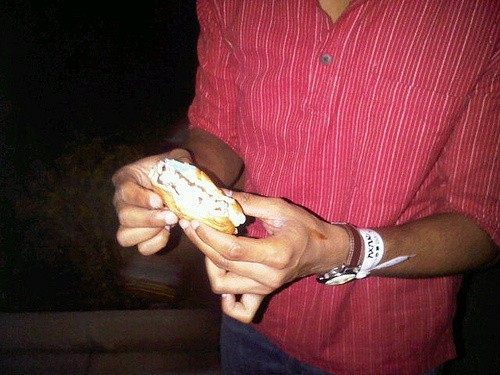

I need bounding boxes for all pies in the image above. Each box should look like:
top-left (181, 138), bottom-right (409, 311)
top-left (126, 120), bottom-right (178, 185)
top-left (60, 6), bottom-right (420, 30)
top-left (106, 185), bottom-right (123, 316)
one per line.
top-left (149, 157), bottom-right (246, 234)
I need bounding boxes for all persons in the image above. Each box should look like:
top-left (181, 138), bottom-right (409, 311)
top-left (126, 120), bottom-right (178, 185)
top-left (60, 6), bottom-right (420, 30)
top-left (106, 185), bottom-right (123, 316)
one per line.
top-left (113, 1), bottom-right (500, 375)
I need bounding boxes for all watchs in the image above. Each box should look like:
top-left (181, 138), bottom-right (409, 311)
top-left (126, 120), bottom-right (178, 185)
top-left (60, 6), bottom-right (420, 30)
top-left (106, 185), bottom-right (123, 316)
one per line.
top-left (317, 220), bottom-right (362, 286)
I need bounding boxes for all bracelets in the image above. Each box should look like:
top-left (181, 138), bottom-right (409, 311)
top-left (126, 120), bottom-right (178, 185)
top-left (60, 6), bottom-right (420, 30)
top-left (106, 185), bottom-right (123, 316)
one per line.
top-left (353, 228), bottom-right (415, 280)
